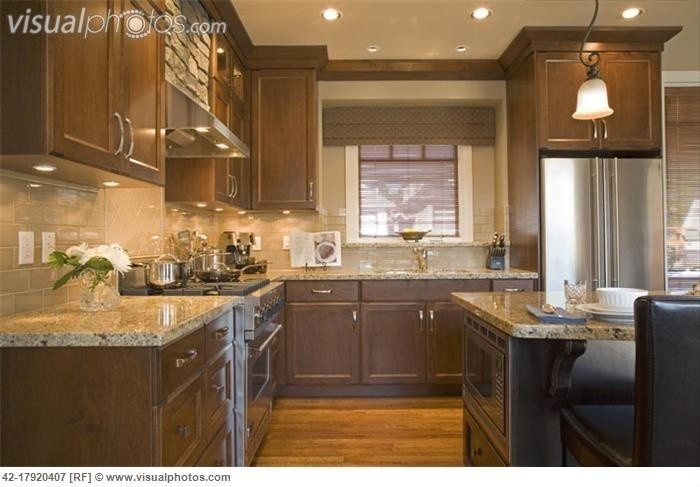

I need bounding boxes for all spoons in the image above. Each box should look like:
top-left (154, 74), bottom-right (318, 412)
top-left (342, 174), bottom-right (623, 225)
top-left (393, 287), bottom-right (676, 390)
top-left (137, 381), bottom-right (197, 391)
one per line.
top-left (542, 303), bottom-right (563, 318)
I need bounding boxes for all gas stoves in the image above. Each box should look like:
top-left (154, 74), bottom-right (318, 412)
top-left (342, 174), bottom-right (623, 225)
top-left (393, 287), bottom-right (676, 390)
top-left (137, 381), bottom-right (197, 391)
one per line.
top-left (122, 277), bottom-right (269, 294)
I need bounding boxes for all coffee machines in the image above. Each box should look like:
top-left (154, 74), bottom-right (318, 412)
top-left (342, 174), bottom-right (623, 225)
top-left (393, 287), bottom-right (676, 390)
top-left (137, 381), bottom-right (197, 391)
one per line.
top-left (219, 230), bottom-right (256, 268)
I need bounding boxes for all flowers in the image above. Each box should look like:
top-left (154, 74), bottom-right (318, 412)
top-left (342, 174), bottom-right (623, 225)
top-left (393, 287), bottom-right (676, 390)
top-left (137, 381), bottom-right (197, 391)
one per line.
top-left (49, 242), bottom-right (132, 291)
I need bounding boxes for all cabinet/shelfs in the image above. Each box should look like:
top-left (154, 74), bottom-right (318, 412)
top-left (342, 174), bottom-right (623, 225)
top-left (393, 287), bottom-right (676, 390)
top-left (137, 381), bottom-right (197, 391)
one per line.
top-left (492, 280), bottom-right (535, 292)
top-left (251, 69), bottom-right (319, 210)
top-left (360, 281), bottom-right (491, 386)
top-left (536, 52), bottom-right (661, 152)
top-left (0, 309), bottom-right (235, 467)
top-left (166, 20), bottom-right (246, 216)
top-left (285, 280), bottom-right (360, 386)
top-left (0, 0), bottom-right (167, 189)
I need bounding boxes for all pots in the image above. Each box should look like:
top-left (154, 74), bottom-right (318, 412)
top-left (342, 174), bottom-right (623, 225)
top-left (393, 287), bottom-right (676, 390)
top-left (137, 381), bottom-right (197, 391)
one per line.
top-left (149, 253), bottom-right (189, 286)
top-left (194, 252), bottom-right (235, 280)
top-left (122, 263), bottom-right (149, 288)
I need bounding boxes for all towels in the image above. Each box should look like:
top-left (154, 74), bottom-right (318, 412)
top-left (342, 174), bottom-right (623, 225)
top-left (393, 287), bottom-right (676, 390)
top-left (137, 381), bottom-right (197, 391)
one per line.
top-left (525, 303), bottom-right (587, 325)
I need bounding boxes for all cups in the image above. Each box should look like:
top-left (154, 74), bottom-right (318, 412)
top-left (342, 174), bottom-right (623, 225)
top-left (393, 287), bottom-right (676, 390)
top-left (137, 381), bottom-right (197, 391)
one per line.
top-left (258, 259), bottom-right (267, 271)
top-left (564, 279), bottom-right (587, 305)
top-left (245, 256), bottom-right (256, 273)
top-left (414, 248), bottom-right (429, 271)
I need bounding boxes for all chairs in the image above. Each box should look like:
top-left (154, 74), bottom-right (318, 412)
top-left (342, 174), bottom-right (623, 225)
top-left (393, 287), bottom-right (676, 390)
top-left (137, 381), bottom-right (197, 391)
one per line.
top-left (559, 295), bottom-right (700, 467)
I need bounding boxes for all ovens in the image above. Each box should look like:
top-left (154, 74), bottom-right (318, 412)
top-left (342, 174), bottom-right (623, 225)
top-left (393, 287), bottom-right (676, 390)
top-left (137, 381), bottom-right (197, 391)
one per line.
top-left (243, 320), bottom-right (283, 464)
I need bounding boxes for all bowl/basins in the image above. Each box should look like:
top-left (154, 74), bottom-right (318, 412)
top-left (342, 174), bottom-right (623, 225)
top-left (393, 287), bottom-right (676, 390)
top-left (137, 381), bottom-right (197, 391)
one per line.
top-left (595, 286), bottom-right (649, 309)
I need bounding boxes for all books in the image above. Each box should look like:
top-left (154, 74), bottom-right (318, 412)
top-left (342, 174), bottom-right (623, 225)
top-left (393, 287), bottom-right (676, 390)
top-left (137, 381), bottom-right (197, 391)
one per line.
top-left (289, 230), bottom-right (343, 268)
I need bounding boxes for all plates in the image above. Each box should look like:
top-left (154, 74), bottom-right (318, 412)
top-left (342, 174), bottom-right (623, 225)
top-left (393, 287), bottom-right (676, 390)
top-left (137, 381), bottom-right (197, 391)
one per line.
top-left (526, 304), bottom-right (593, 322)
top-left (575, 303), bottom-right (635, 323)
top-left (395, 229), bottom-right (432, 242)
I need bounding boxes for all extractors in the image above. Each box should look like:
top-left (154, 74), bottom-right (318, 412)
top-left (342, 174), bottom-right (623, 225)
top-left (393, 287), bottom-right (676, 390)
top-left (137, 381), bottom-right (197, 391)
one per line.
top-left (164, 78), bottom-right (251, 158)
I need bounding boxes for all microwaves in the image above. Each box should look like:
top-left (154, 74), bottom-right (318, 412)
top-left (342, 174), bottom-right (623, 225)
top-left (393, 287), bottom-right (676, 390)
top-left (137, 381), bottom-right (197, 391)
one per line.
top-left (462, 308), bottom-right (511, 464)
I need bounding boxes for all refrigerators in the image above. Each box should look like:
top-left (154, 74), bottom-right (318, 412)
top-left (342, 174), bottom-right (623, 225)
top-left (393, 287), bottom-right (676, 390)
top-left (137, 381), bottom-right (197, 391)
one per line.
top-left (541, 157), bottom-right (667, 291)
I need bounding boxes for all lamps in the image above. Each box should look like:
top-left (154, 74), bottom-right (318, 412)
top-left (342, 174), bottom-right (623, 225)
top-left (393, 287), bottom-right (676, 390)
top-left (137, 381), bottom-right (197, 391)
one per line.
top-left (572, 0), bottom-right (615, 120)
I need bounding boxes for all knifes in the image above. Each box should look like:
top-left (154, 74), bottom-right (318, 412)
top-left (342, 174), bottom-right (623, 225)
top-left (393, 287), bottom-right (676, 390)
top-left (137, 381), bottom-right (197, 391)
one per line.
top-left (487, 233), bottom-right (506, 257)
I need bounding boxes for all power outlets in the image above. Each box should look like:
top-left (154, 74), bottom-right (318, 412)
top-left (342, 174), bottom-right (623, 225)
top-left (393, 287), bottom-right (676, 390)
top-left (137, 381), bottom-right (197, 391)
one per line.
top-left (251, 237), bottom-right (261, 251)
top-left (41, 231), bottom-right (56, 264)
top-left (282, 236), bottom-right (289, 250)
top-left (18, 231), bottom-right (35, 265)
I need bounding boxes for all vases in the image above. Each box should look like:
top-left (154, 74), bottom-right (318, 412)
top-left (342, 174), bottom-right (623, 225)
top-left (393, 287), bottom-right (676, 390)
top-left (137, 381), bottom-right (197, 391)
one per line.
top-left (80, 275), bottom-right (121, 312)
